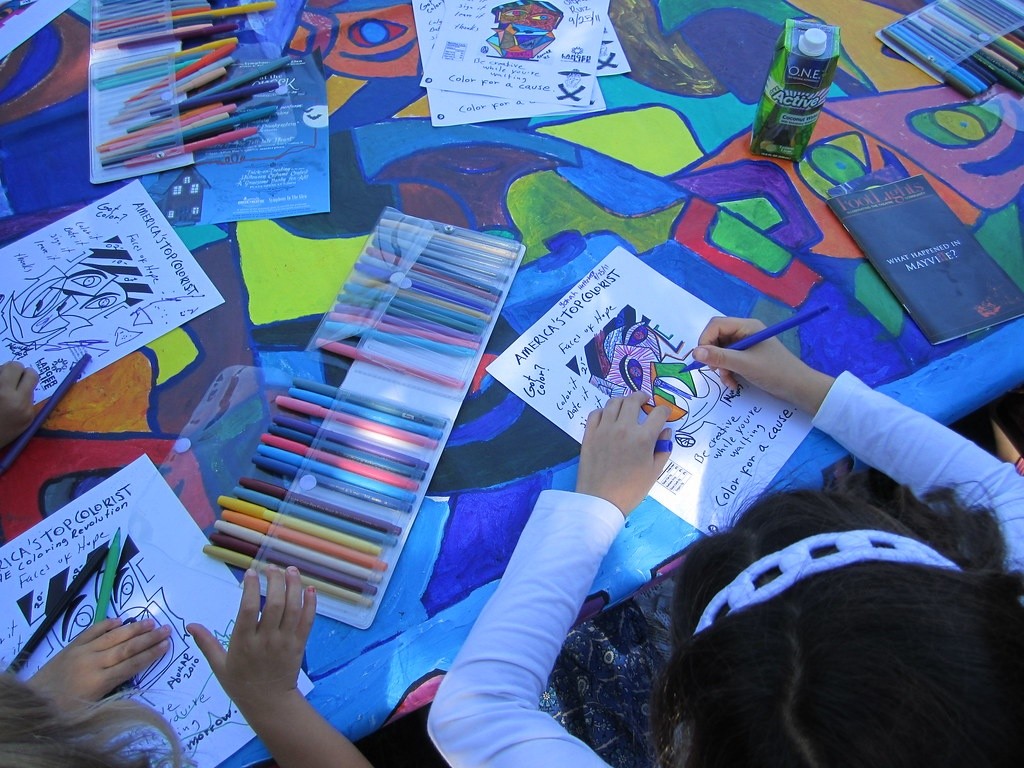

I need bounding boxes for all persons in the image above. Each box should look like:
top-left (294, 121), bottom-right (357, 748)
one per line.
top-left (426, 316), bottom-right (1023, 768)
top-left (0, 361), bottom-right (39, 452)
top-left (1, 565), bottom-right (368, 768)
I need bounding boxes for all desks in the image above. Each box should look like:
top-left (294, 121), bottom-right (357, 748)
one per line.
top-left (0, 0), bottom-right (1024, 768)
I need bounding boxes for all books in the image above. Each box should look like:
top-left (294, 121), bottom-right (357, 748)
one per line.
top-left (826, 172), bottom-right (1024, 346)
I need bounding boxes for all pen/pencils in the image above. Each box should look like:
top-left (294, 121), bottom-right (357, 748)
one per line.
top-left (217, 494), bottom-right (383, 557)
top-left (91, 0), bottom-right (292, 169)
top-left (202, 544), bottom-right (374, 608)
top-left (678, 303), bottom-right (831, 374)
top-left (221, 510), bottom-right (389, 572)
top-left (255, 443), bottom-right (417, 504)
top-left (0, 353), bottom-right (92, 477)
top-left (231, 486), bottom-right (399, 547)
top-left (93, 526), bottom-right (121, 623)
top-left (213, 519), bottom-right (384, 583)
top-left (324, 210), bottom-right (521, 358)
top-left (275, 377), bottom-right (447, 450)
top-left (315, 337), bottom-right (466, 390)
top-left (251, 454), bottom-right (413, 513)
top-left (268, 425), bottom-right (427, 481)
top-left (208, 532), bottom-right (378, 596)
top-left (881, 0), bottom-right (1024, 99)
top-left (271, 413), bottom-right (430, 470)
top-left (6, 545), bottom-right (110, 673)
top-left (238, 476), bottom-right (403, 536)
top-left (260, 433), bottom-right (420, 491)
